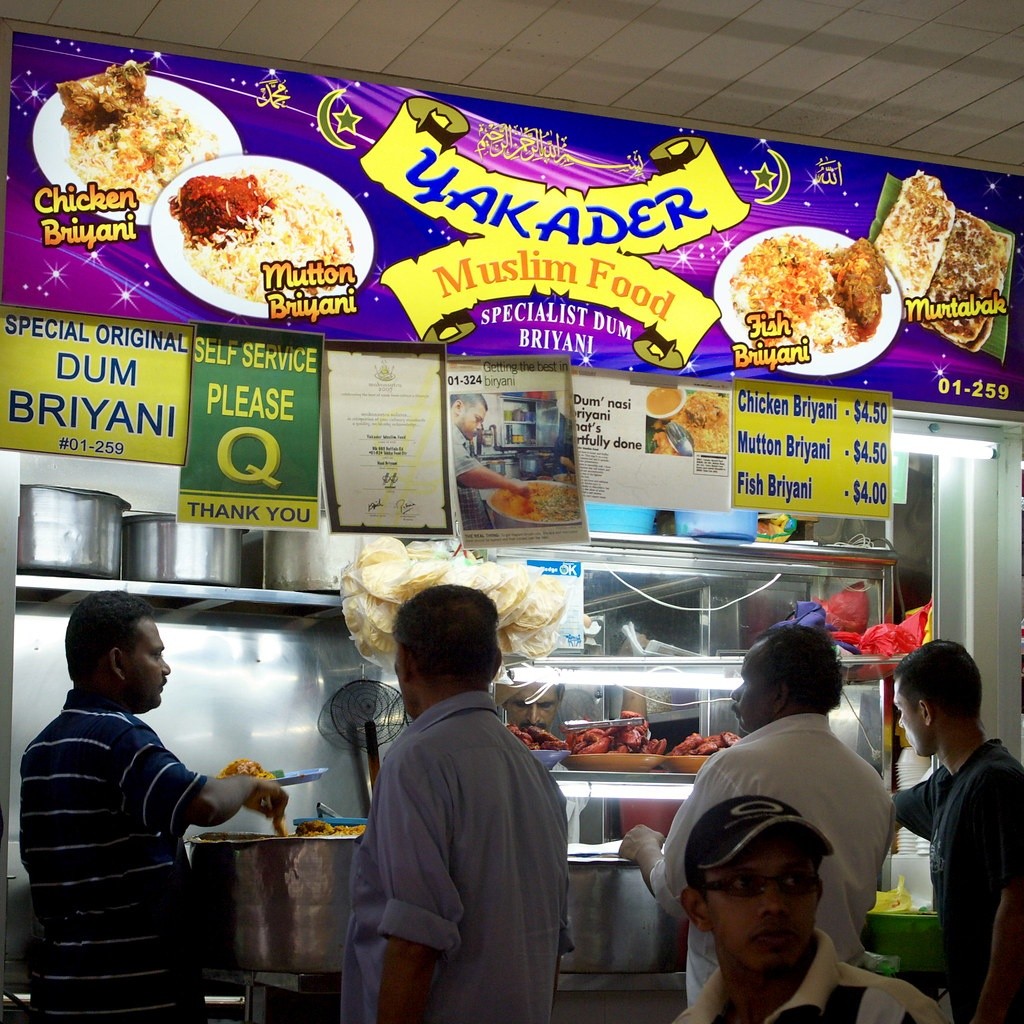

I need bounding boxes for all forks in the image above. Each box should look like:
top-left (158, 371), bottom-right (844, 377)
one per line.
top-left (666, 422), bottom-right (694, 458)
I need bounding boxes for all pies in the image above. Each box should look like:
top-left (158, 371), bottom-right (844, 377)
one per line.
top-left (874, 171), bottom-right (1012, 353)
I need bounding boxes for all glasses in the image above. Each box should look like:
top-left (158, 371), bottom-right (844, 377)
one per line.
top-left (706, 871), bottom-right (819, 897)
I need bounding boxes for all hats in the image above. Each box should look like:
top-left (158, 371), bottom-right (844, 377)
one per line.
top-left (685, 795), bottom-right (834, 870)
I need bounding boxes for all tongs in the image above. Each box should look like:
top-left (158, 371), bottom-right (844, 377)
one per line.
top-left (316, 802), bottom-right (344, 818)
top-left (559, 718), bottom-right (644, 732)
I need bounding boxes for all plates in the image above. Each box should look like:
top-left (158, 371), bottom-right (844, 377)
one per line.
top-left (263, 765), bottom-right (327, 792)
top-left (658, 753), bottom-right (711, 774)
top-left (150, 155), bottom-right (374, 319)
top-left (33, 74), bottom-right (244, 226)
top-left (714, 225), bottom-right (901, 376)
top-left (560, 753), bottom-right (667, 772)
top-left (530, 750), bottom-right (570, 771)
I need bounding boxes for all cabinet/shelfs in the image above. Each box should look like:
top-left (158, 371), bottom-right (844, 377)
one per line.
top-left (498, 395), bottom-right (560, 450)
top-left (473, 534), bottom-right (894, 801)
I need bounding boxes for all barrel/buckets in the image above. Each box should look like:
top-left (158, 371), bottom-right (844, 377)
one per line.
top-left (188, 830), bottom-right (355, 971)
top-left (263, 511), bottom-right (364, 590)
top-left (560, 854), bottom-right (687, 975)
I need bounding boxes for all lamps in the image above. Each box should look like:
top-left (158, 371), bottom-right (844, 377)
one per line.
top-left (506, 664), bottom-right (746, 691)
top-left (551, 779), bottom-right (695, 800)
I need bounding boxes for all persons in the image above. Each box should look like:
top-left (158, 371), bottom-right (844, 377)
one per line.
top-left (554, 436), bottom-right (578, 475)
top-left (503, 632), bottom-right (652, 742)
top-left (617, 621), bottom-right (1024, 1024)
top-left (17, 590), bottom-right (290, 1024)
top-left (449, 393), bottom-right (533, 530)
top-left (340, 584), bottom-right (576, 1024)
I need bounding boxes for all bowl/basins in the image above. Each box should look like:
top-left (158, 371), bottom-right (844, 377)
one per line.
top-left (646, 387), bottom-right (688, 420)
top-left (17, 482), bottom-right (132, 577)
top-left (121, 513), bottom-right (251, 585)
top-left (484, 479), bottom-right (582, 529)
top-left (893, 747), bottom-right (940, 855)
top-left (866, 910), bottom-right (949, 974)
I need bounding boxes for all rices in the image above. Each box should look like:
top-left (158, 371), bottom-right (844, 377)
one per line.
top-left (186, 170), bottom-right (353, 306)
top-left (731, 231), bottom-right (861, 356)
top-left (70, 99), bottom-right (220, 207)
